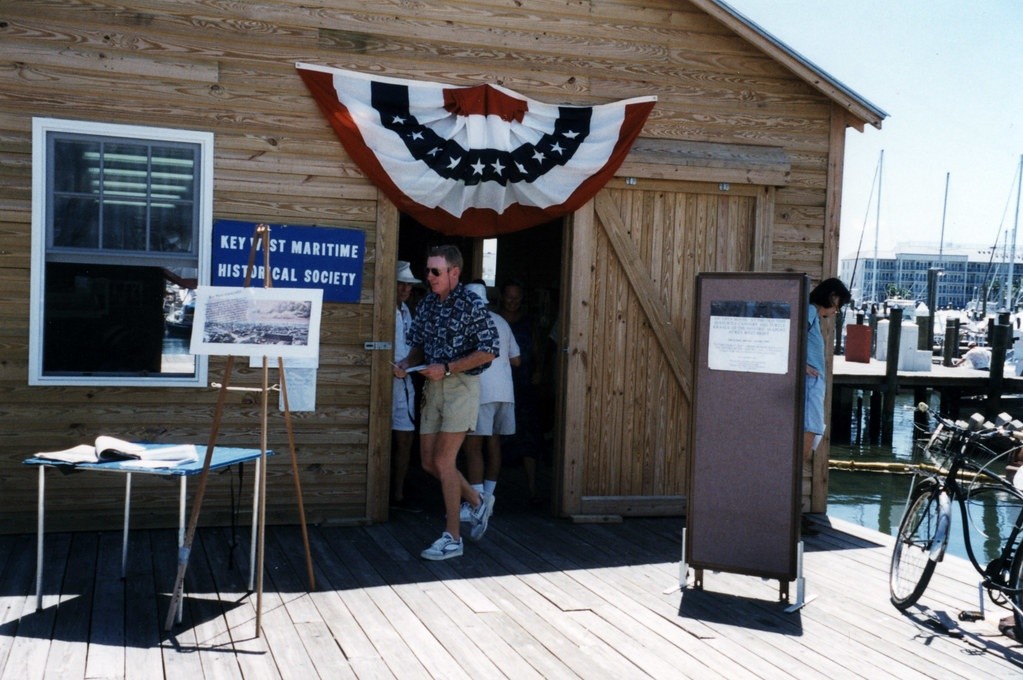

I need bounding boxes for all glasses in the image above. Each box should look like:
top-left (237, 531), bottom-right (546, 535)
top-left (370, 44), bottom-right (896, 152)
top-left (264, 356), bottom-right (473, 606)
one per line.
top-left (831, 300), bottom-right (840, 315)
top-left (426, 267), bottom-right (453, 276)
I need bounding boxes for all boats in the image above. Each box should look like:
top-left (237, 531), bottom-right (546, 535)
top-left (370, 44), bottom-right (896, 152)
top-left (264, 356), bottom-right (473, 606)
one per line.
top-left (164, 290), bottom-right (197, 331)
top-left (831, 148), bottom-right (1023, 356)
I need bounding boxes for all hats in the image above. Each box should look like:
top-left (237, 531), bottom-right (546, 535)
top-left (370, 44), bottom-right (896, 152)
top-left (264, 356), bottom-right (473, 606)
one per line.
top-left (465, 283), bottom-right (489, 304)
top-left (396, 261), bottom-right (422, 283)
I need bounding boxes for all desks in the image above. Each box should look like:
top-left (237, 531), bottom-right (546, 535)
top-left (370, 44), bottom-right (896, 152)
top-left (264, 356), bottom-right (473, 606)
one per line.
top-left (23, 442), bottom-right (276, 624)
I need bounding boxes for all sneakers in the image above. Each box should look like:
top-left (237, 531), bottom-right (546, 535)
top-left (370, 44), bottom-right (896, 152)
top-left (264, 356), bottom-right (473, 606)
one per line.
top-left (470, 492), bottom-right (496, 540)
top-left (447, 501), bottom-right (471, 521)
top-left (421, 532), bottom-right (463, 560)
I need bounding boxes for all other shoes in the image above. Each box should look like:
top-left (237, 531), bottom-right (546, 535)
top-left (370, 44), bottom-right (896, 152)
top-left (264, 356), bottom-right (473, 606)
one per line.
top-left (529, 494), bottom-right (550, 505)
top-left (391, 498), bottom-right (424, 512)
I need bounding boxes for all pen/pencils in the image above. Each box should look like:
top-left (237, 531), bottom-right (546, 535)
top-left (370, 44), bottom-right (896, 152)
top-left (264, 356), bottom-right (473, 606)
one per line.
top-left (388, 360), bottom-right (399, 368)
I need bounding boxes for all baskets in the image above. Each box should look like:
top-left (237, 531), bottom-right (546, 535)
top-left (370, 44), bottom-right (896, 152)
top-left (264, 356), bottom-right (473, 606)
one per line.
top-left (910, 442), bottom-right (954, 480)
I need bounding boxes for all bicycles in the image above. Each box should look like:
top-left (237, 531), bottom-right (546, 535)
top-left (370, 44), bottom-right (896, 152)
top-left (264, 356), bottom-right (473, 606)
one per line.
top-left (887, 402), bottom-right (1023, 643)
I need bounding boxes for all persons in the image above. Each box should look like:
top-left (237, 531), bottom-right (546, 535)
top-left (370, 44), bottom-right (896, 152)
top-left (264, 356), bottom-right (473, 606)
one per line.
top-left (860, 301), bottom-right (868, 317)
top-left (801, 277), bottom-right (854, 537)
top-left (390, 246), bottom-right (561, 561)
top-left (953, 341), bottom-right (991, 371)
top-left (966, 309), bottom-right (984, 322)
